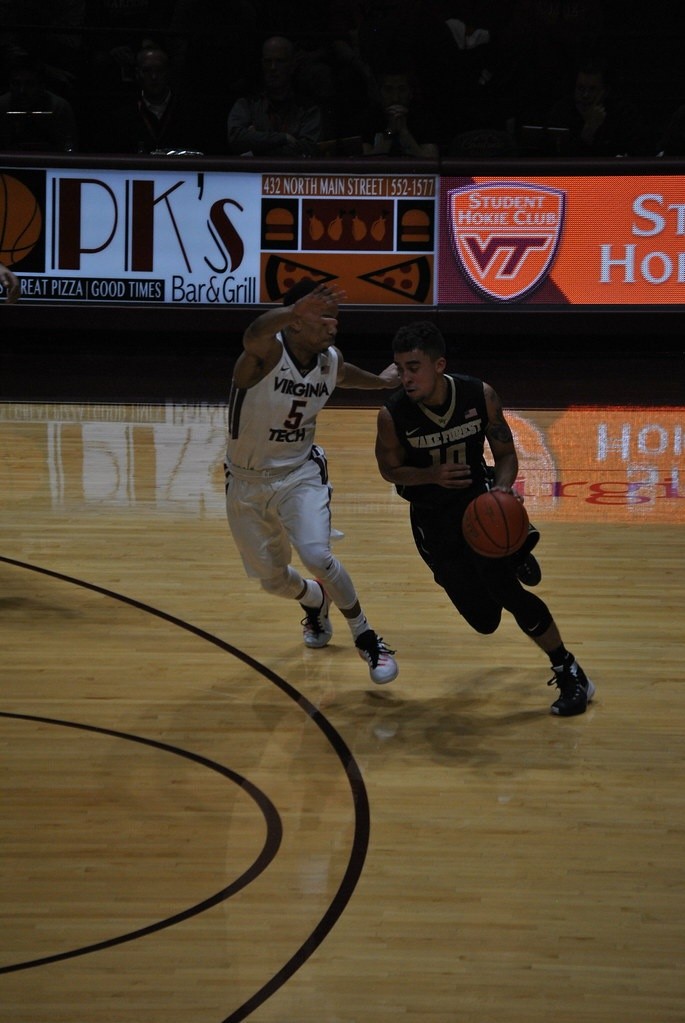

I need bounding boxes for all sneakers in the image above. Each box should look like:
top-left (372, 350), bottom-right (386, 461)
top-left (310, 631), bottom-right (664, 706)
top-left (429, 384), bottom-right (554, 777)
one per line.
top-left (516, 551), bottom-right (540, 587)
top-left (548, 653), bottom-right (595, 716)
top-left (353, 628), bottom-right (399, 684)
top-left (300, 576), bottom-right (333, 647)
top-left (301, 646), bottom-right (335, 706)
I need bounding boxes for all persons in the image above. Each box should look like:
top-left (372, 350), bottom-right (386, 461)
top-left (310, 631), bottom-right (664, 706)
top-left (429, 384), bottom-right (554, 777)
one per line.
top-left (0, 0), bottom-right (685, 159)
top-left (0, 263), bottom-right (22, 304)
top-left (221, 276), bottom-right (402, 685)
top-left (376, 321), bottom-right (596, 718)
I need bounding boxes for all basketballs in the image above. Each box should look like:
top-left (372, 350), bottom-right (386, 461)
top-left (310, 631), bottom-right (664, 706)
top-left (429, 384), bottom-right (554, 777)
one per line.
top-left (462, 491), bottom-right (531, 560)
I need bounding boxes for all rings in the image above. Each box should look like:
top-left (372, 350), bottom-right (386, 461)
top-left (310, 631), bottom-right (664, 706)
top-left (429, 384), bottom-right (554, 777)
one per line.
top-left (0, 275), bottom-right (7, 282)
